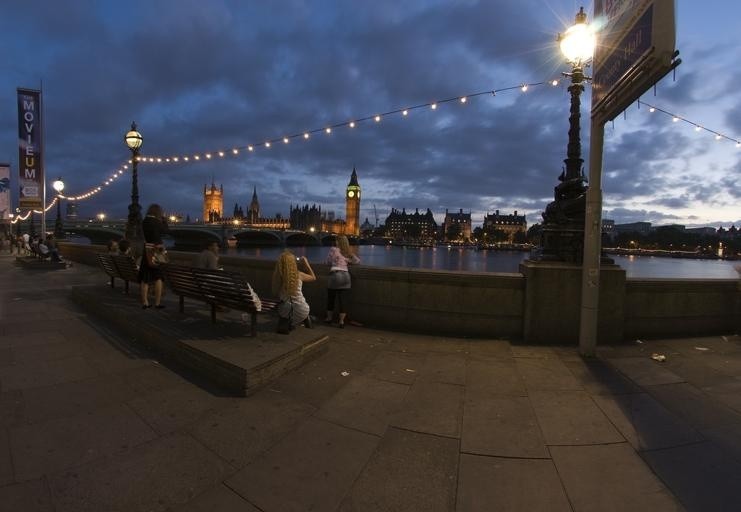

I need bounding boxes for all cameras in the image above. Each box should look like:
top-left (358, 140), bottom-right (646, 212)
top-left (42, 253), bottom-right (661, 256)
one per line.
top-left (296, 257), bottom-right (301, 261)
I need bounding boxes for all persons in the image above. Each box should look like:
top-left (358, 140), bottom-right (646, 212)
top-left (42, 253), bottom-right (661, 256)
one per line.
top-left (5, 230), bottom-right (62, 261)
top-left (272, 251), bottom-right (317, 334)
top-left (323, 234), bottom-right (361, 329)
top-left (200, 238), bottom-right (224, 270)
top-left (108, 204), bottom-right (169, 310)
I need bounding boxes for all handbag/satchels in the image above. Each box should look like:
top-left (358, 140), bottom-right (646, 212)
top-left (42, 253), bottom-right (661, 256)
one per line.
top-left (277, 317), bottom-right (291, 335)
top-left (145, 242), bottom-right (170, 266)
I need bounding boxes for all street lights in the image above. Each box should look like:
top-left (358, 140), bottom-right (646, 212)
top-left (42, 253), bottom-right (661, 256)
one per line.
top-left (124, 119), bottom-right (143, 246)
top-left (539, 6), bottom-right (596, 260)
top-left (16, 208), bottom-right (21, 237)
top-left (52, 175), bottom-right (65, 238)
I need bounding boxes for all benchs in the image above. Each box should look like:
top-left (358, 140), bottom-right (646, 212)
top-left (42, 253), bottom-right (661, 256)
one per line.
top-left (97, 254), bottom-right (282, 341)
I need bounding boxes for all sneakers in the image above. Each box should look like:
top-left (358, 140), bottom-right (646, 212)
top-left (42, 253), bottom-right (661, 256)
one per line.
top-left (321, 319), bottom-right (345, 328)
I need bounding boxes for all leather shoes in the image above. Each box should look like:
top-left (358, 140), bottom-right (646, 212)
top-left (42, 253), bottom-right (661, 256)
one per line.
top-left (155, 304), bottom-right (167, 309)
top-left (142, 304), bottom-right (151, 310)
